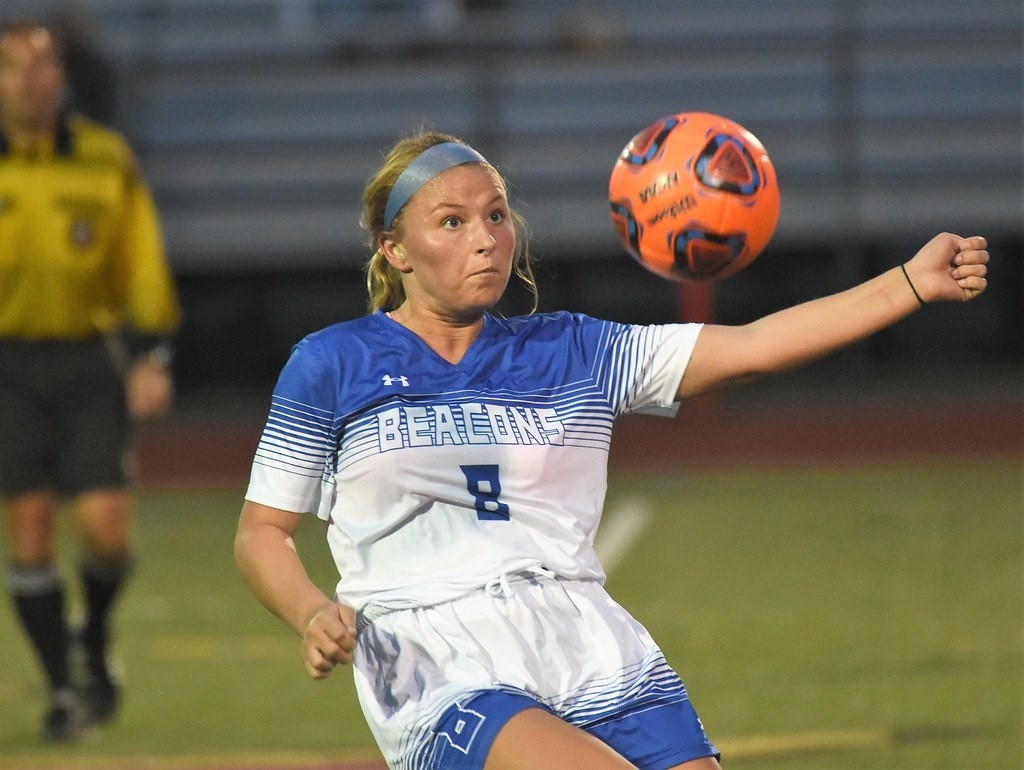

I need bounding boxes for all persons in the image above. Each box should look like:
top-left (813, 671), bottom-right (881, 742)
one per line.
top-left (1, 21), bottom-right (181, 747)
top-left (235, 132), bottom-right (989, 770)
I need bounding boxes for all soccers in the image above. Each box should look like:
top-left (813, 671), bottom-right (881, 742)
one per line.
top-left (607, 110), bottom-right (782, 287)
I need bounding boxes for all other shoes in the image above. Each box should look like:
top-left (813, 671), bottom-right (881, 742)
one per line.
top-left (79, 625), bottom-right (117, 720)
top-left (44, 676), bottom-right (69, 739)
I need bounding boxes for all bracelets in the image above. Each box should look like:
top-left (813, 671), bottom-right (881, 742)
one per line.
top-left (901, 264), bottom-right (926, 305)
